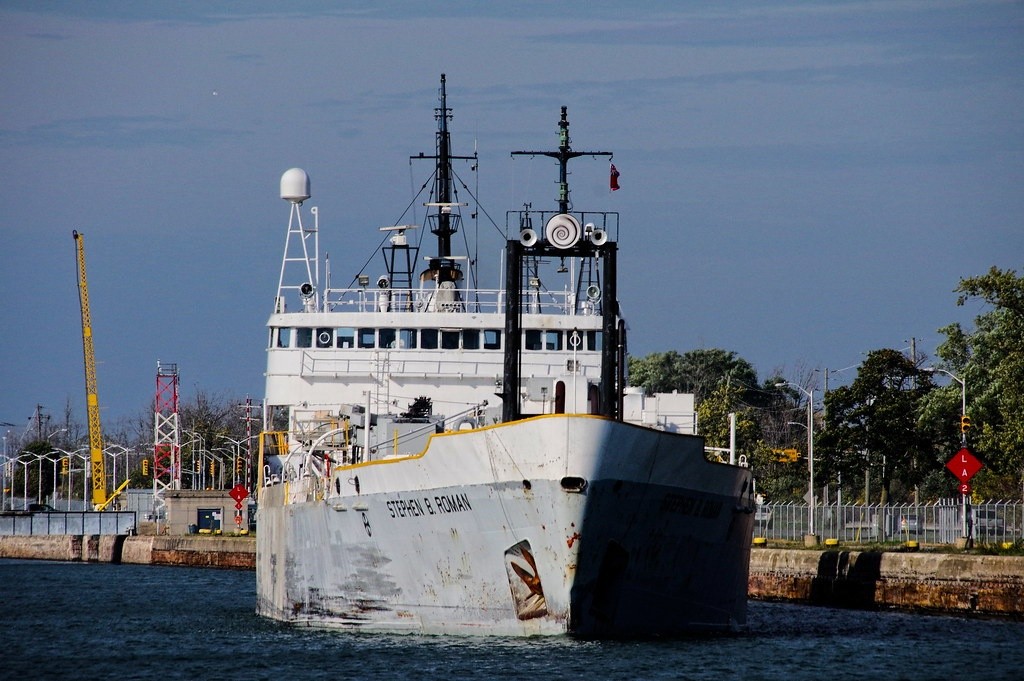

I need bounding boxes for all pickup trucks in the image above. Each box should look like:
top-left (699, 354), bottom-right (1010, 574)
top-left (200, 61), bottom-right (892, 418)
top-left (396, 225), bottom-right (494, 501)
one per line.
top-left (971, 509), bottom-right (1004, 535)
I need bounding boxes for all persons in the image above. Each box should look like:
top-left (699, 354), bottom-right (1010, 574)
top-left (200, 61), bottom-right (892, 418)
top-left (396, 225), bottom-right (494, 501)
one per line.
top-left (902, 515), bottom-right (907, 531)
top-left (162, 526), bottom-right (166, 535)
top-left (209, 519), bottom-right (215, 529)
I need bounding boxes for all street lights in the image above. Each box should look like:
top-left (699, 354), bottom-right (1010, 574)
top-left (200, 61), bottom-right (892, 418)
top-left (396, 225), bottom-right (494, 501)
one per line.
top-left (0, 435), bottom-right (260, 510)
top-left (788, 405), bottom-right (822, 491)
top-left (923, 367), bottom-right (971, 538)
top-left (47, 429), bottom-right (68, 444)
top-left (776, 382), bottom-right (813, 534)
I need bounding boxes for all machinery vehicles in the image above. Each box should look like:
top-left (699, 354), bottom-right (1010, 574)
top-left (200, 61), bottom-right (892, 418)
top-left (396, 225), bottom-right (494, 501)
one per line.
top-left (72, 229), bottom-right (109, 512)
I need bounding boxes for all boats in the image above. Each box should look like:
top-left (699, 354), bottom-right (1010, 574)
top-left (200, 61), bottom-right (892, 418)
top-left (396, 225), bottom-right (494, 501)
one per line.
top-left (252, 74), bottom-right (757, 636)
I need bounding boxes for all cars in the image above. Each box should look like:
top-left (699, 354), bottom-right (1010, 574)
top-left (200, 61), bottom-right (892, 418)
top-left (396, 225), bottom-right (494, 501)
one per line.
top-left (753, 506), bottom-right (773, 529)
top-left (897, 514), bottom-right (922, 535)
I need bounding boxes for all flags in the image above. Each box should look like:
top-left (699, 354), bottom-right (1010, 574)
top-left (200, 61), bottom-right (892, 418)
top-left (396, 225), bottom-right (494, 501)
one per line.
top-left (609, 164), bottom-right (620, 191)
